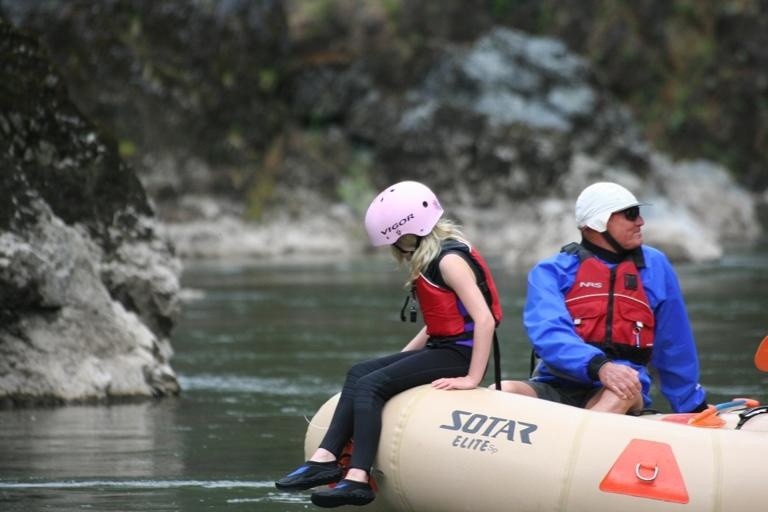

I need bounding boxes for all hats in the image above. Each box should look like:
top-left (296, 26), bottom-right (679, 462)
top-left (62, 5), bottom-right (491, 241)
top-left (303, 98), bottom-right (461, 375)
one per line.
top-left (575, 182), bottom-right (653, 233)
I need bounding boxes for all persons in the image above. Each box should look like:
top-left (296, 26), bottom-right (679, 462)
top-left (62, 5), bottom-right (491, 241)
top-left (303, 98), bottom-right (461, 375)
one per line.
top-left (486, 180), bottom-right (708, 417)
top-left (272, 179), bottom-right (504, 508)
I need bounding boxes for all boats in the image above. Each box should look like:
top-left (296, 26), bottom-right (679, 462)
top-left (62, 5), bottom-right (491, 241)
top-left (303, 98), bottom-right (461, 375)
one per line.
top-left (303, 379), bottom-right (768, 512)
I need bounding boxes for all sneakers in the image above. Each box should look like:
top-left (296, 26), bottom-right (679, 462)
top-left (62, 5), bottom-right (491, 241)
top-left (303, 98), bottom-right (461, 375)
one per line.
top-left (275, 460), bottom-right (344, 493)
top-left (311, 478), bottom-right (376, 508)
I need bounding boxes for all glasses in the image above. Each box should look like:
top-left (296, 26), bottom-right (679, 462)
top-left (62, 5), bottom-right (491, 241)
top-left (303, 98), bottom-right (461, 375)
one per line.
top-left (612, 206), bottom-right (639, 222)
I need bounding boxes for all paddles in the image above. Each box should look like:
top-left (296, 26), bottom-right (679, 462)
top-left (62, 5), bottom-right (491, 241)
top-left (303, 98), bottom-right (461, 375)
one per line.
top-left (662, 398), bottom-right (759, 429)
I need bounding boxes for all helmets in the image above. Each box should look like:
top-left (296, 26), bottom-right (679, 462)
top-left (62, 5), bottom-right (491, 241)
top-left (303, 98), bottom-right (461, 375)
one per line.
top-left (364, 181), bottom-right (444, 249)
